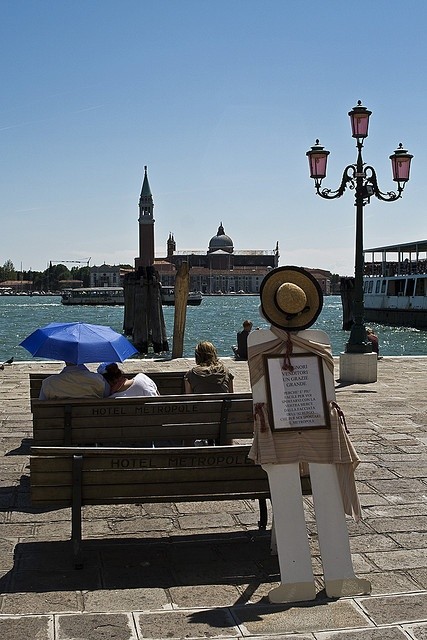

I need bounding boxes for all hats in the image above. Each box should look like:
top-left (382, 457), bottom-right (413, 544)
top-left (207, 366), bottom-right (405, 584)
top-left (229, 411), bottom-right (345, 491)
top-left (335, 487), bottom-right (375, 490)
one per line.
top-left (259, 266), bottom-right (323, 331)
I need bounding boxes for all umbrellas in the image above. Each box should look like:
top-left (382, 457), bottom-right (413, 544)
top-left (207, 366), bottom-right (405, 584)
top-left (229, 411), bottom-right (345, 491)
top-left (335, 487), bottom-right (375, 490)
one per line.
top-left (18, 320), bottom-right (139, 365)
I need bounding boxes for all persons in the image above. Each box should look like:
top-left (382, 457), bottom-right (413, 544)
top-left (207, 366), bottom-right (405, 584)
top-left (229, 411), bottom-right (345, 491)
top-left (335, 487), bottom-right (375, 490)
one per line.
top-left (183, 341), bottom-right (233, 394)
top-left (37, 362), bottom-right (111, 400)
top-left (102, 363), bottom-right (159, 398)
top-left (230, 320), bottom-right (253, 361)
top-left (365, 328), bottom-right (378, 354)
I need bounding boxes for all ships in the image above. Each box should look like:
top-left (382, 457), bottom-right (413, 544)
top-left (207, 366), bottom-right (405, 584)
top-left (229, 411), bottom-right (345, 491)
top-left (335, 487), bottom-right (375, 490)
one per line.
top-left (60, 282), bottom-right (204, 306)
top-left (362, 240), bottom-right (427, 328)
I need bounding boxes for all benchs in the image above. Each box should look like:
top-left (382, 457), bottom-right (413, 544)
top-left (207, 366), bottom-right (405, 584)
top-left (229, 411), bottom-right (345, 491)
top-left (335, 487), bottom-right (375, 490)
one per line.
top-left (30, 392), bottom-right (254, 448)
top-left (31, 445), bottom-right (309, 536)
top-left (28, 372), bottom-right (234, 398)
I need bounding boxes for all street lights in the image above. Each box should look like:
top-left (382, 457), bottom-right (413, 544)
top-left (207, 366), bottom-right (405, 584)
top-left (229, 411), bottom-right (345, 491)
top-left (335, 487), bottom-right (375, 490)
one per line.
top-left (306, 99), bottom-right (413, 354)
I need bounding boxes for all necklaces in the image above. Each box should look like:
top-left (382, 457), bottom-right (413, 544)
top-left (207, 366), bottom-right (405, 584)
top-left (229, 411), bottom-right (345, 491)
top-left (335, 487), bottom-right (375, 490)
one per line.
top-left (116, 380), bottom-right (126, 393)
